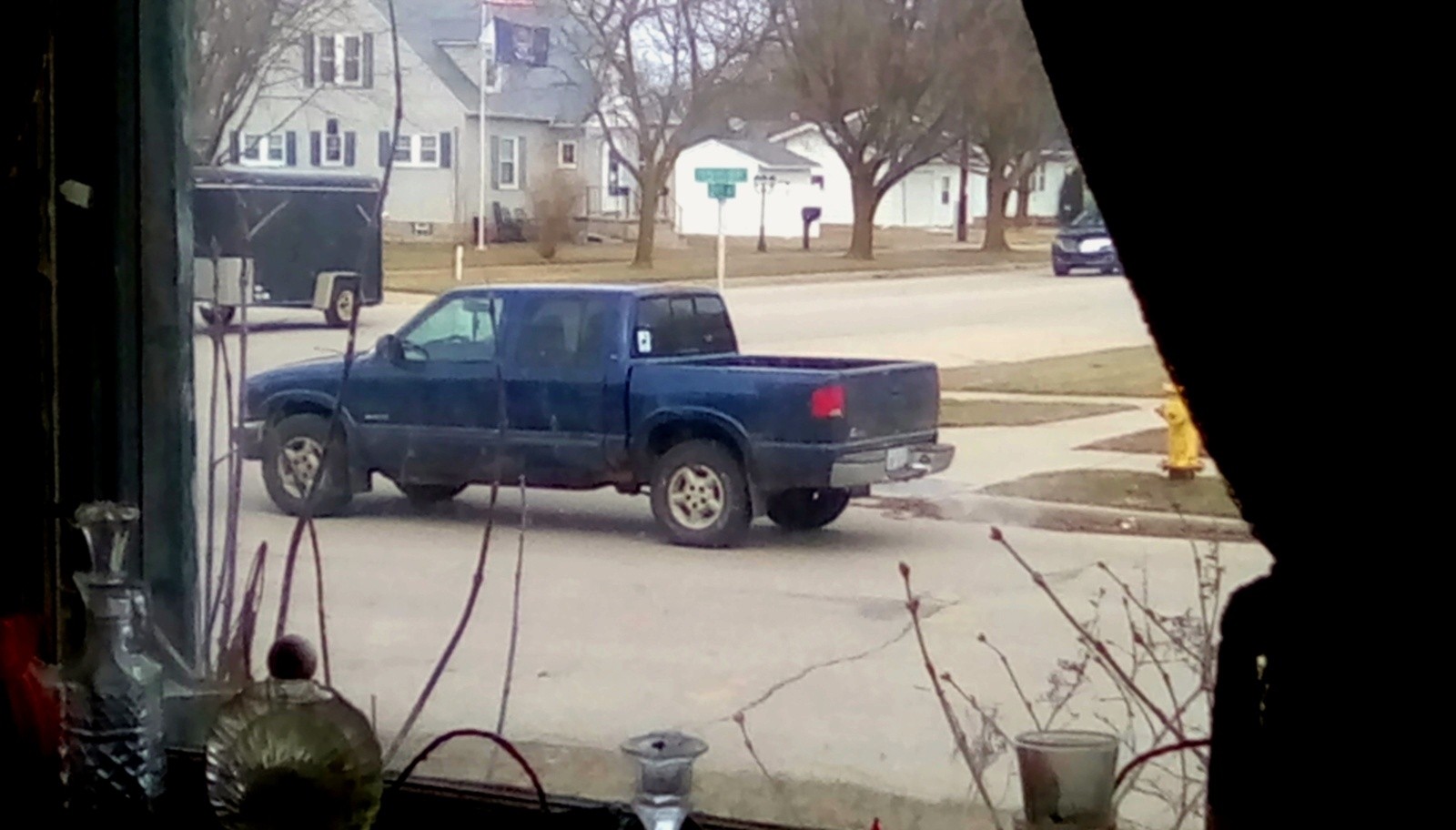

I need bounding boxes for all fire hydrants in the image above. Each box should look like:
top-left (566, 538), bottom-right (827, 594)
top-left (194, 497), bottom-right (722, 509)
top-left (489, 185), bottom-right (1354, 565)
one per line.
top-left (1154, 381), bottom-right (1210, 481)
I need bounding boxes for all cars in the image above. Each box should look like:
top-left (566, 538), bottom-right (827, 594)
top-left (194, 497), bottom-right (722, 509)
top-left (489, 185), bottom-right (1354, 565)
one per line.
top-left (1050, 203), bottom-right (1124, 278)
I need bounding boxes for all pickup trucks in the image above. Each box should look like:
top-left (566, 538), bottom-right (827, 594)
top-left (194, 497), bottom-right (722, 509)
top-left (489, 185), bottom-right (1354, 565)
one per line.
top-left (232, 285), bottom-right (956, 549)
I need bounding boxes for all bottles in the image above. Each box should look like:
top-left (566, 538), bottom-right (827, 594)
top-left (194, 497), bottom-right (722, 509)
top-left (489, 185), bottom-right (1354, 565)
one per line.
top-left (66, 501), bottom-right (166, 802)
top-left (1012, 730), bottom-right (1119, 830)
top-left (204, 633), bottom-right (383, 830)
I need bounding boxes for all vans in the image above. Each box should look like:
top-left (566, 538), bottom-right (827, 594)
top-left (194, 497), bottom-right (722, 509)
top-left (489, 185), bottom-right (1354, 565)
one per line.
top-left (189, 167), bottom-right (384, 329)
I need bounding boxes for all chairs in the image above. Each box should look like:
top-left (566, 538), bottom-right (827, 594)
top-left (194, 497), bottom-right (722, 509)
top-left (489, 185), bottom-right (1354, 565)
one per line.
top-left (522, 316), bottom-right (567, 365)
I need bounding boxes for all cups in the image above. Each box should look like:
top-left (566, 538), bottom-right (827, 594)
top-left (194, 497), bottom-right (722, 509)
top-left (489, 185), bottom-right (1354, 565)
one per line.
top-left (621, 732), bottom-right (709, 830)
